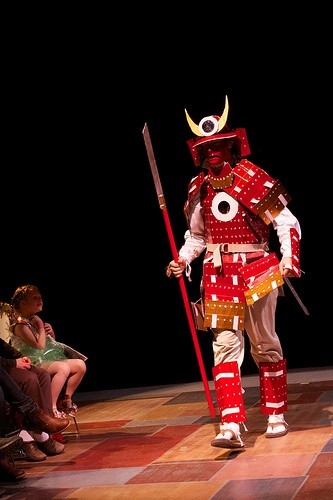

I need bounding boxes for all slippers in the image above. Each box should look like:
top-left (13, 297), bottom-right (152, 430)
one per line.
top-left (211, 429), bottom-right (245, 448)
top-left (265, 423), bottom-right (289, 438)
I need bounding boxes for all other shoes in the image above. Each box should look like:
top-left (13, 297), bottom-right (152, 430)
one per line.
top-left (0, 455), bottom-right (27, 482)
top-left (5, 429), bottom-right (47, 462)
top-left (0, 435), bottom-right (23, 458)
top-left (35, 438), bottom-right (65, 455)
top-left (48, 433), bottom-right (67, 443)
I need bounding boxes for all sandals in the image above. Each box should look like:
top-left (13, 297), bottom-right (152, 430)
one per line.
top-left (59, 399), bottom-right (78, 417)
top-left (48, 405), bottom-right (66, 420)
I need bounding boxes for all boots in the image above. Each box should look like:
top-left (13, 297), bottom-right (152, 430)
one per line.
top-left (12, 396), bottom-right (71, 435)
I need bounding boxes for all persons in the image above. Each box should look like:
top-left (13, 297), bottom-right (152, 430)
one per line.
top-left (0, 338), bottom-right (70, 481)
top-left (166, 115), bottom-right (302, 449)
top-left (8, 285), bottom-right (87, 420)
